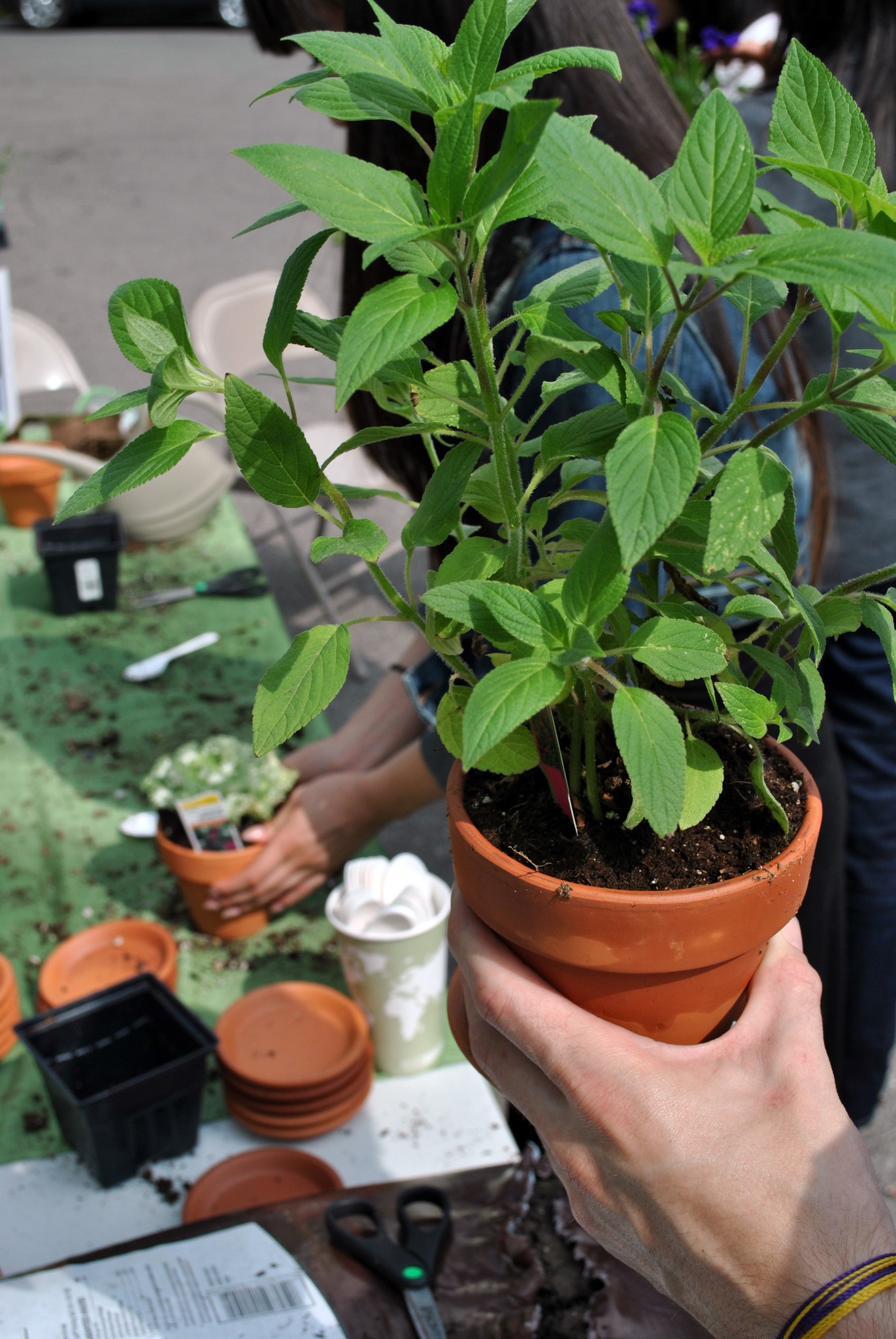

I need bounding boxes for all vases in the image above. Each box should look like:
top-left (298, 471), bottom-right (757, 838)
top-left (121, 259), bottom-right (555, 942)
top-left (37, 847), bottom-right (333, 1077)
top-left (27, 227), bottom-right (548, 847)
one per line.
top-left (12, 974), bottom-right (221, 1191)
top-left (0, 453), bottom-right (61, 529)
top-left (35, 511), bottom-right (125, 616)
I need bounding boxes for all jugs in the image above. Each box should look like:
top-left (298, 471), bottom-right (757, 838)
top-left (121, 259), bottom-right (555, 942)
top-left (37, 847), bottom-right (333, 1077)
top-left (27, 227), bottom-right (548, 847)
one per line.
top-left (0, 393), bottom-right (243, 543)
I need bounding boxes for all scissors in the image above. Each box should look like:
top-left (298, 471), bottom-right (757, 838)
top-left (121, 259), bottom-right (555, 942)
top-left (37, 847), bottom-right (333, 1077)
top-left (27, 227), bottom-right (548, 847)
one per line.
top-left (129, 563), bottom-right (270, 608)
top-left (326, 1183), bottom-right (451, 1339)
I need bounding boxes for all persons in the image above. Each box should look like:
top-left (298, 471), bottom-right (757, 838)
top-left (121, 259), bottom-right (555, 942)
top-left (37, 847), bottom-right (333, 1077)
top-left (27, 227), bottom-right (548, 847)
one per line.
top-left (447, 887), bottom-right (896, 1339)
top-left (202, 0), bottom-right (896, 1130)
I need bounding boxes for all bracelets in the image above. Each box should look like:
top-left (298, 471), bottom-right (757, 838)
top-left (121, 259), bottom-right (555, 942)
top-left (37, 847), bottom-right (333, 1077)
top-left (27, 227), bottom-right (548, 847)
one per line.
top-left (390, 663), bottom-right (407, 673)
top-left (775, 1252), bottom-right (896, 1339)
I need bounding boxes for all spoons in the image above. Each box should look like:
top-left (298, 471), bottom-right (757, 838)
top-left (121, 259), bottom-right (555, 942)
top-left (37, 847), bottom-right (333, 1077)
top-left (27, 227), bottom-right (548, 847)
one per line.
top-left (121, 632), bottom-right (221, 682)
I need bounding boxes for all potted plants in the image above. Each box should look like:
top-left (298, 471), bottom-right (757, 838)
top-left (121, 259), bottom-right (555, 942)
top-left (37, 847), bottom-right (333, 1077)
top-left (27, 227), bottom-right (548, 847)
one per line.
top-left (50, 0), bottom-right (896, 1042)
top-left (137, 735), bottom-right (299, 936)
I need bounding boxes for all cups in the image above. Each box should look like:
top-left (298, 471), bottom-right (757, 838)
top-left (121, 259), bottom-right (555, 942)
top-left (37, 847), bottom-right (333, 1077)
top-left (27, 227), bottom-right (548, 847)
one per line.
top-left (323, 871), bottom-right (453, 1079)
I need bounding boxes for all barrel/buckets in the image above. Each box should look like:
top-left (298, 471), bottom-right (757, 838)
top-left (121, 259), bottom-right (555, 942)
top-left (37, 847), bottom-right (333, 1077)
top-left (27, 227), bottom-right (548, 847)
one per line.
top-left (0, 443), bottom-right (67, 528)
top-left (34, 510), bottom-right (120, 617)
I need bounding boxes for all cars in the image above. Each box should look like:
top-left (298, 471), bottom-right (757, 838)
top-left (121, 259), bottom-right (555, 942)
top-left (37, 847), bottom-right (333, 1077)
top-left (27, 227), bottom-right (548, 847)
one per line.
top-left (16, 0), bottom-right (248, 34)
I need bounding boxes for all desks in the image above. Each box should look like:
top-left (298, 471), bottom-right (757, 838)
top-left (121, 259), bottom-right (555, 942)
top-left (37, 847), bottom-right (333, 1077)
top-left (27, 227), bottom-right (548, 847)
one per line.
top-left (0, 419), bottom-right (522, 1277)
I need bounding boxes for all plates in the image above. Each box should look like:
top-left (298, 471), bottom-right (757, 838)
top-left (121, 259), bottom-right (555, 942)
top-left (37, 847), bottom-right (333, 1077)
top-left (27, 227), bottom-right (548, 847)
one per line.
top-left (39, 920), bottom-right (177, 1015)
top-left (0, 953), bottom-right (20, 1056)
top-left (218, 983), bottom-right (375, 1139)
top-left (179, 1149), bottom-right (345, 1224)
